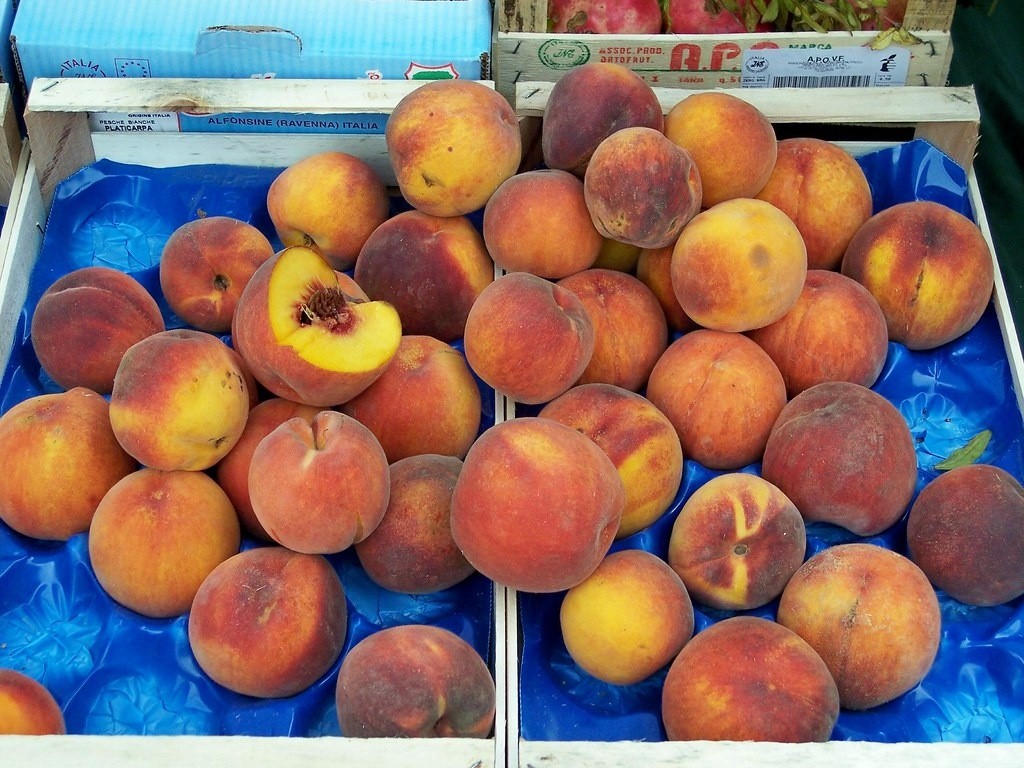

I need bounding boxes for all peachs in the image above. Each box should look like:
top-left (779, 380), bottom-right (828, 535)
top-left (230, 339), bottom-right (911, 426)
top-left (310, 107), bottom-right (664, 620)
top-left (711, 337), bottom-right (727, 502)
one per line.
top-left (1, 64), bottom-right (1023, 740)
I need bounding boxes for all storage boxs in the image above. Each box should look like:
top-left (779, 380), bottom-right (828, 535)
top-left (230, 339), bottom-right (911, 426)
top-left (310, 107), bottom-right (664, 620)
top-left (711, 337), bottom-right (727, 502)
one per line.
top-left (0, 0), bottom-right (1024, 768)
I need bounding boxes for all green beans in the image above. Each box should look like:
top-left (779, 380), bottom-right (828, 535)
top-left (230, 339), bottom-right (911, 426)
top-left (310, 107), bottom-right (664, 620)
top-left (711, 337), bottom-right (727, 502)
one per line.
top-left (721, 0), bottom-right (888, 31)
